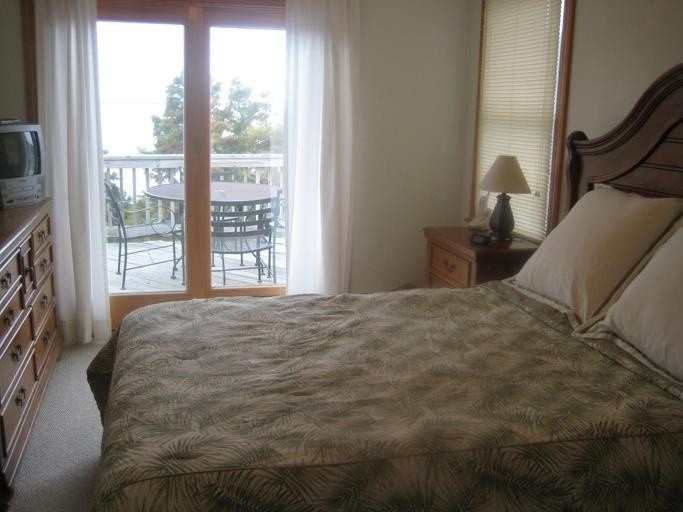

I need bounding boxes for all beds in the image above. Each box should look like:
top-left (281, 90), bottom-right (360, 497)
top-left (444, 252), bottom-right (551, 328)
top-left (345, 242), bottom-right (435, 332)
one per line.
top-left (84, 62), bottom-right (683, 512)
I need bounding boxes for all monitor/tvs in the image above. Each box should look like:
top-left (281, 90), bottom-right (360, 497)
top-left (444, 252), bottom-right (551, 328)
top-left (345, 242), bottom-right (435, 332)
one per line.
top-left (0, 123), bottom-right (49, 212)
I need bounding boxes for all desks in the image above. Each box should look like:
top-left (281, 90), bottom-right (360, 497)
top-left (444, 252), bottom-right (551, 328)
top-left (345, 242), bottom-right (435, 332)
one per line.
top-left (143, 182), bottom-right (278, 255)
top-left (0, 197), bottom-right (63, 489)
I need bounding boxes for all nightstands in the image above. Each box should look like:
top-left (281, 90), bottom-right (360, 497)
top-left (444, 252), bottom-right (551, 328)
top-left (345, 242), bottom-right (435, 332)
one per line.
top-left (422, 225), bottom-right (543, 290)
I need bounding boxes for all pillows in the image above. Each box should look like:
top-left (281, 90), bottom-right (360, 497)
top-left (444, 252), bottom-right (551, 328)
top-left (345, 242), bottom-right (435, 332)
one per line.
top-left (571, 216), bottom-right (683, 386)
top-left (501, 184), bottom-right (683, 331)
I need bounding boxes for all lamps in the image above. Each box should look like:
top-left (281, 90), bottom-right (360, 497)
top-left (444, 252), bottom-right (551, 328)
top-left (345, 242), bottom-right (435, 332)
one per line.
top-left (478, 155), bottom-right (531, 238)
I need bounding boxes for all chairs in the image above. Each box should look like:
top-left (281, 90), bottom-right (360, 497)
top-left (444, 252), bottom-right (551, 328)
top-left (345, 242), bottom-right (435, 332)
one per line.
top-left (172, 189), bottom-right (280, 285)
top-left (104, 166), bottom-right (184, 286)
